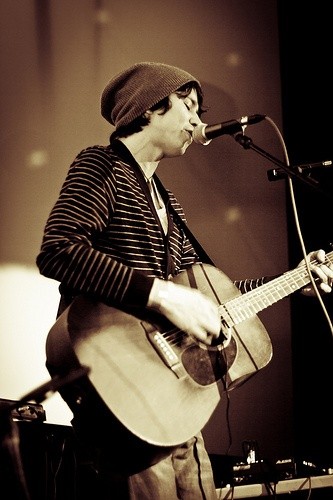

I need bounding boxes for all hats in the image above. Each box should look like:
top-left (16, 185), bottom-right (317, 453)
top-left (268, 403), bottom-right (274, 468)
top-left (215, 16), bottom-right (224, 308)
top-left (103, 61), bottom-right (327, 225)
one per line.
top-left (100, 62), bottom-right (202, 128)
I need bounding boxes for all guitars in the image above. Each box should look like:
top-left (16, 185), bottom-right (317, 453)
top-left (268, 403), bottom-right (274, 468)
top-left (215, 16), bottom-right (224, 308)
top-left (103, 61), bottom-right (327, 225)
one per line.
top-left (44, 251), bottom-right (333, 449)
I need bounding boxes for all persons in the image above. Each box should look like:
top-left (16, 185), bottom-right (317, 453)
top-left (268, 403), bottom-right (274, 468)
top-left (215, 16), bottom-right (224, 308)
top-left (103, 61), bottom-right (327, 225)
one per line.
top-left (36, 62), bottom-right (333, 500)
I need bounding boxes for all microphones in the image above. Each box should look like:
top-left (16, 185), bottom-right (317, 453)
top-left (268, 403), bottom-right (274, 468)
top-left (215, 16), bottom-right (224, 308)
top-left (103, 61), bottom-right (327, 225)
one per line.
top-left (191, 113), bottom-right (265, 147)
